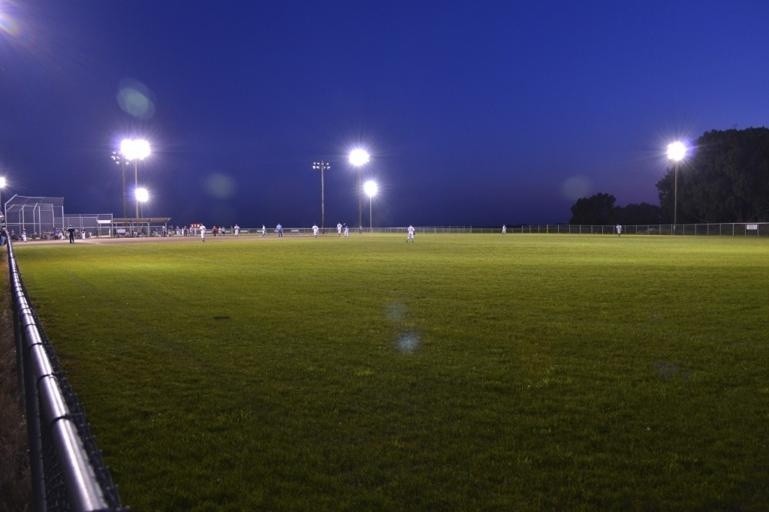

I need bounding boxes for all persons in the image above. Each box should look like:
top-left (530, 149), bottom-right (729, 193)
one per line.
top-left (502, 224), bottom-right (507, 235)
top-left (112, 222), bottom-right (241, 242)
top-left (53, 226), bottom-right (61, 240)
top-left (336, 221), bottom-right (342, 237)
top-left (262, 224), bottom-right (265, 235)
top-left (408, 224), bottom-right (416, 244)
top-left (343, 224), bottom-right (349, 238)
top-left (616, 223), bottom-right (622, 237)
top-left (312, 223), bottom-right (319, 238)
top-left (67, 229), bottom-right (75, 244)
top-left (276, 222), bottom-right (282, 237)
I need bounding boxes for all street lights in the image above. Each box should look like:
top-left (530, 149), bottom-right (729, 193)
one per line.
top-left (349, 148), bottom-right (377, 229)
top-left (110, 137), bottom-right (151, 218)
top-left (311, 159), bottom-right (331, 231)
